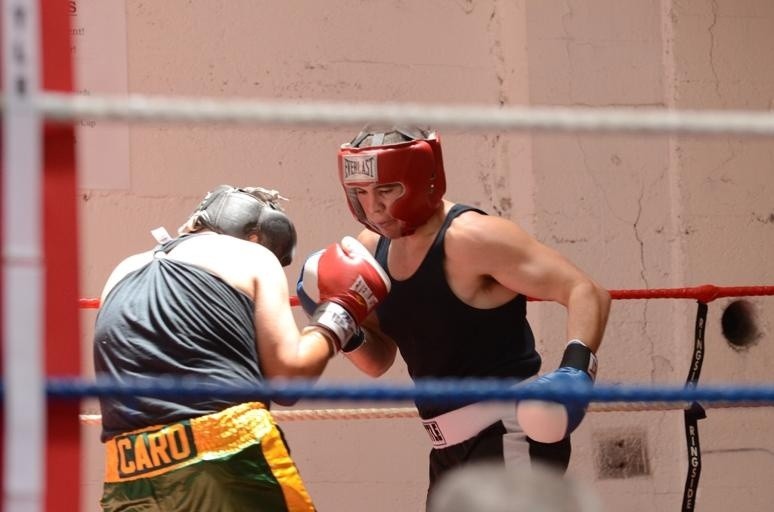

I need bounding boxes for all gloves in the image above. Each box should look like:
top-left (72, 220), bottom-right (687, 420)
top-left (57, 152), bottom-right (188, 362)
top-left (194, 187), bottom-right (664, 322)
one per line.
top-left (296, 236), bottom-right (392, 354)
top-left (515, 340), bottom-right (598, 444)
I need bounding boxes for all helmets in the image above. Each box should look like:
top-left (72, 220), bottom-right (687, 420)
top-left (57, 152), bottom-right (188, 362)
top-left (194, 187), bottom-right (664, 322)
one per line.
top-left (338, 126), bottom-right (446, 239)
top-left (190, 184), bottom-right (297, 267)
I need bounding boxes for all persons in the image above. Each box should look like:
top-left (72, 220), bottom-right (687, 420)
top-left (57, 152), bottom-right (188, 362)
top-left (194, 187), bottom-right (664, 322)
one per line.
top-left (92, 185), bottom-right (392, 512)
top-left (296, 126), bottom-right (612, 512)
